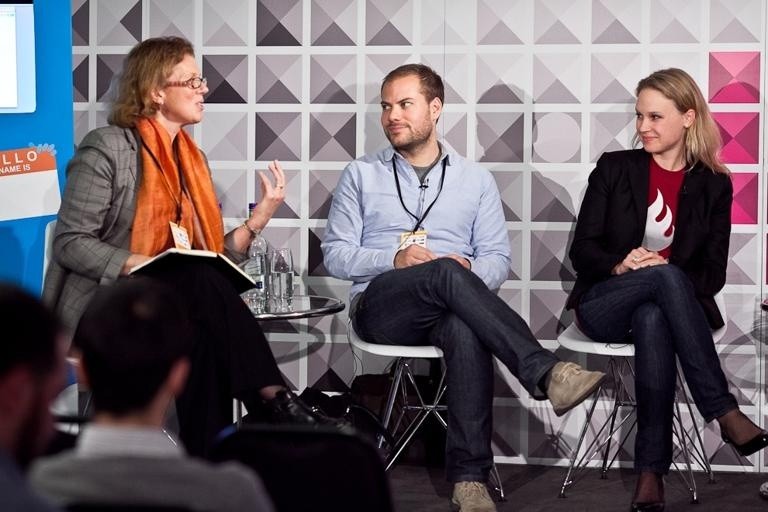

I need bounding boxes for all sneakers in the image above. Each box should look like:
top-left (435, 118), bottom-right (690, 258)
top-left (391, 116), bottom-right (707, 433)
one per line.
top-left (542, 363), bottom-right (607, 416)
top-left (449, 481), bottom-right (498, 511)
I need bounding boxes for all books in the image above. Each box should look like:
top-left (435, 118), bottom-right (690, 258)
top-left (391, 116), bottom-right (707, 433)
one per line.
top-left (128, 246), bottom-right (258, 298)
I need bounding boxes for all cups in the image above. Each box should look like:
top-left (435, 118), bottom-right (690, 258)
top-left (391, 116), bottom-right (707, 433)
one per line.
top-left (241, 298), bottom-right (292, 316)
top-left (235, 239), bottom-right (297, 297)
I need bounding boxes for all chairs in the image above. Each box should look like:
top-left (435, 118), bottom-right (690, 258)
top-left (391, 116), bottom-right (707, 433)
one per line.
top-left (41, 220), bottom-right (177, 447)
top-left (556, 295), bottom-right (728, 502)
top-left (346, 318), bottom-right (506, 499)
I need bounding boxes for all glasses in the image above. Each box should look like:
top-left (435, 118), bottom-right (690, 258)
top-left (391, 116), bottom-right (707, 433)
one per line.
top-left (164, 77), bottom-right (208, 89)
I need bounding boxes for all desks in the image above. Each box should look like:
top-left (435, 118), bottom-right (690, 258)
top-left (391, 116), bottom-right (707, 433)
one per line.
top-left (236, 294), bottom-right (345, 429)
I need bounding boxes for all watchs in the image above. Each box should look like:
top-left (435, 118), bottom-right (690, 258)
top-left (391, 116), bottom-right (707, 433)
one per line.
top-left (242, 219), bottom-right (261, 239)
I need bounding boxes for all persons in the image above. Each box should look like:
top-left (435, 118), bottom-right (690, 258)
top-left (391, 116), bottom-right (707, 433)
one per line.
top-left (42, 35), bottom-right (287, 413)
top-left (320, 62), bottom-right (607, 512)
top-left (566, 66), bottom-right (768, 512)
top-left (25, 285), bottom-right (272, 511)
top-left (0, 279), bottom-right (68, 512)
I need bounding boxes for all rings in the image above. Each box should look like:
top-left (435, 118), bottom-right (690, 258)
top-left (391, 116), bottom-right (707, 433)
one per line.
top-left (632, 256), bottom-right (638, 263)
top-left (275, 183), bottom-right (283, 190)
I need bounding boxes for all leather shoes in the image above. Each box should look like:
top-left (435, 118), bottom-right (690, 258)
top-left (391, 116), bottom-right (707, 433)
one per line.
top-left (721, 416), bottom-right (767, 455)
top-left (253, 393), bottom-right (348, 430)
top-left (630, 470), bottom-right (666, 508)
